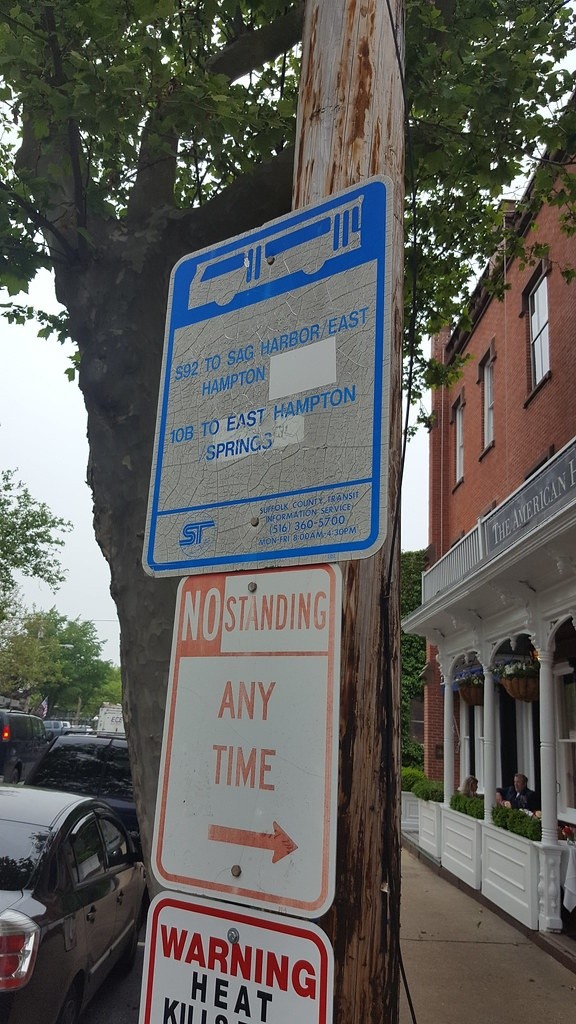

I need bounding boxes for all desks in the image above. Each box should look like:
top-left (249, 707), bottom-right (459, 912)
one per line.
top-left (558, 839), bottom-right (576, 914)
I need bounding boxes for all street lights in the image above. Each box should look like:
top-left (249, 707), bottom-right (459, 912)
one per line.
top-left (22, 644), bottom-right (74, 713)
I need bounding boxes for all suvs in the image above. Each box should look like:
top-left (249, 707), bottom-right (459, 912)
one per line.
top-left (0, 704), bottom-right (52, 786)
top-left (23, 730), bottom-right (143, 856)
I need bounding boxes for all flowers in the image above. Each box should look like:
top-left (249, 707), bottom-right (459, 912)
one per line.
top-left (561, 826), bottom-right (576, 836)
top-left (489, 653), bottom-right (541, 683)
top-left (454, 667), bottom-right (485, 688)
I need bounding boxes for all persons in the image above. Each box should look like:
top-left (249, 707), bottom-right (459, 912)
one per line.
top-left (495, 773), bottom-right (541, 812)
top-left (462, 776), bottom-right (484, 800)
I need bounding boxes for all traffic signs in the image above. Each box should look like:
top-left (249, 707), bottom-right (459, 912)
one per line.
top-left (148, 564), bottom-right (345, 920)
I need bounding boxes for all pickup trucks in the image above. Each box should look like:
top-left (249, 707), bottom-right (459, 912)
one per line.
top-left (43, 721), bottom-right (86, 738)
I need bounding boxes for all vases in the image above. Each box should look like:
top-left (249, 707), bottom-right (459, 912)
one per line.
top-left (567, 836), bottom-right (576, 847)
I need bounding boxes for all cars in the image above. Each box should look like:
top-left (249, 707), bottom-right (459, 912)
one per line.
top-left (80, 725), bottom-right (93, 734)
top-left (0, 782), bottom-right (146, 1024)
